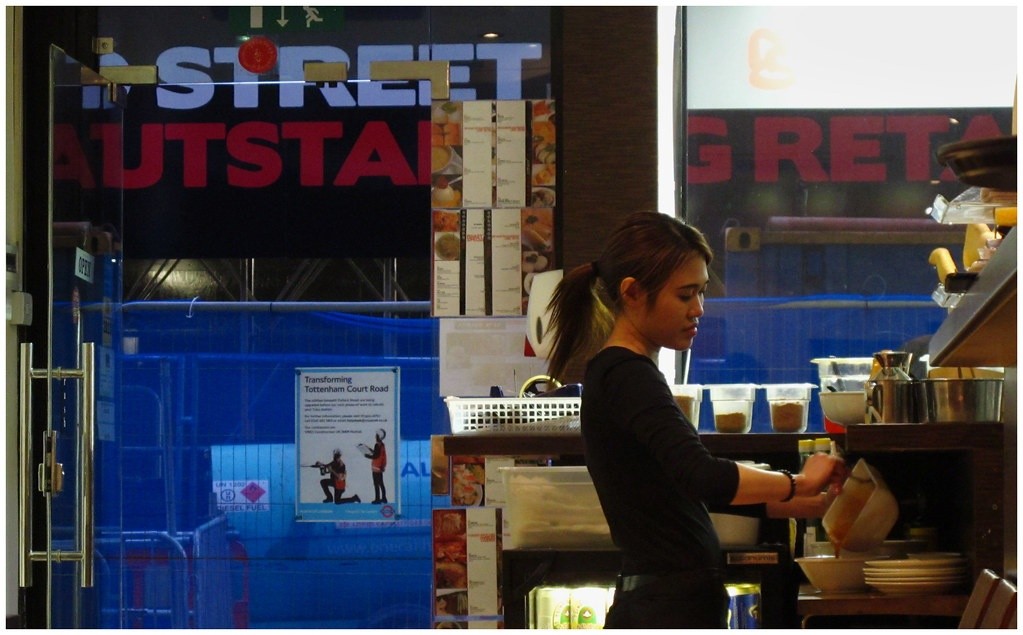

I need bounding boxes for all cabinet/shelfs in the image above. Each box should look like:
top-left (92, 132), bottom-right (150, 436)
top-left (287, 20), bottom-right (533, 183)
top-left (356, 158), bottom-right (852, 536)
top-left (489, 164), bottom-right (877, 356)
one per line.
top-left (435, 220), bottom-right (1016, 629)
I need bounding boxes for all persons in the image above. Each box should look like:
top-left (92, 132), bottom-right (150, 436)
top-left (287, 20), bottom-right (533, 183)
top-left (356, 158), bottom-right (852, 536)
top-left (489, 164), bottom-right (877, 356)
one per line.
top-left (538, 211), bottom-right (843, 631)
top-left (363, 429), bottom-right (389, 506)
top-left (314, 449), bottom-right (362, 505)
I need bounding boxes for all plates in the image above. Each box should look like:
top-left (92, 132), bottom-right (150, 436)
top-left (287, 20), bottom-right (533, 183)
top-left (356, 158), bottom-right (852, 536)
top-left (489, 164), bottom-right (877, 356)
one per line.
top-left (862, 551), bottom-right (973, 593)
top-left (533, 187), bottom-right (556, 206)
top-left (432, 146), bottom-right (452, 173)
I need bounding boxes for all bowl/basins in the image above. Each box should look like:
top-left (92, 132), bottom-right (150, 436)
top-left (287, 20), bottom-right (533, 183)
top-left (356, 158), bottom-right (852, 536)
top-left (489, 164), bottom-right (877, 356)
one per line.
top-left (795, 554), bottom-right (891, 592)
top-left (919, 378), bottom-right (1003, 423)
top-left (908, 527), bottom-right (936, 539)
top-left (822, 458), bottom-right (899, 550)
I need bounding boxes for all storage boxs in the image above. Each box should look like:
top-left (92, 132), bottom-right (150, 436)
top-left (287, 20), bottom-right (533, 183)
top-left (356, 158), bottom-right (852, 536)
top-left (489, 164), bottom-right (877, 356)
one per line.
top-left (498, 467), bottom-right (617, 550)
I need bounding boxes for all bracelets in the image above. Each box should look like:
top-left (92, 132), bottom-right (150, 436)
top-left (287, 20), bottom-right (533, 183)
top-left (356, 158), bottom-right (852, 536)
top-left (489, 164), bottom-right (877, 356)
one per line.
top-left (777, 470), bottom-right (796, 504)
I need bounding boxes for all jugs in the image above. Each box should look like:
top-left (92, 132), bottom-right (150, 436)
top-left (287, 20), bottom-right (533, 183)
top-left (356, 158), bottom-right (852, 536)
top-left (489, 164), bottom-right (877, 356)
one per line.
top-left (867, 351), bottom-right (915, 423)
